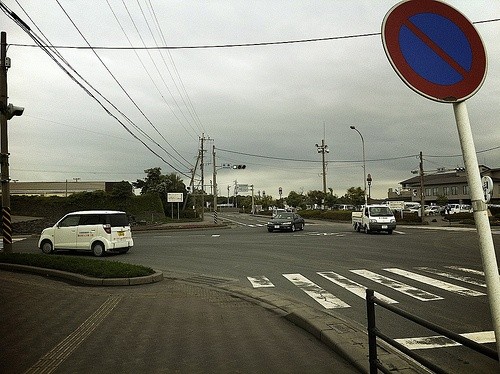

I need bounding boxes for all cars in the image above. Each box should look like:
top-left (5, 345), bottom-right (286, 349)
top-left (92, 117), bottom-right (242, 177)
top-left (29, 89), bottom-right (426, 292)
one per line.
top-left (267, 212), bottom-right (305, 232)
top-left (404, 202), bottom-right (471, 217)
top-left (37, 210), bottom-right (134, 257)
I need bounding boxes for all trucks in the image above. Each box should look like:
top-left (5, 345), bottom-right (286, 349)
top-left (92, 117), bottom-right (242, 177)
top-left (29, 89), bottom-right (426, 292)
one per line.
top-left (351, 205), bottom-right (396, 234)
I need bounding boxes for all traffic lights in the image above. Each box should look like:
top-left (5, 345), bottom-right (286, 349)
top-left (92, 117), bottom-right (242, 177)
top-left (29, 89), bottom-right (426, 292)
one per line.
top-left (8, 103), bottom-right (26, 121)
top-left (233, 165), bottom-right (247, 170)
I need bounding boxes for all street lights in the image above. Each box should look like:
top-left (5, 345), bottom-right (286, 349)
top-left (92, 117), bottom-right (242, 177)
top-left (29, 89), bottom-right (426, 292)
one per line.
top-left (349, 125), bottom-right (368, 205)
